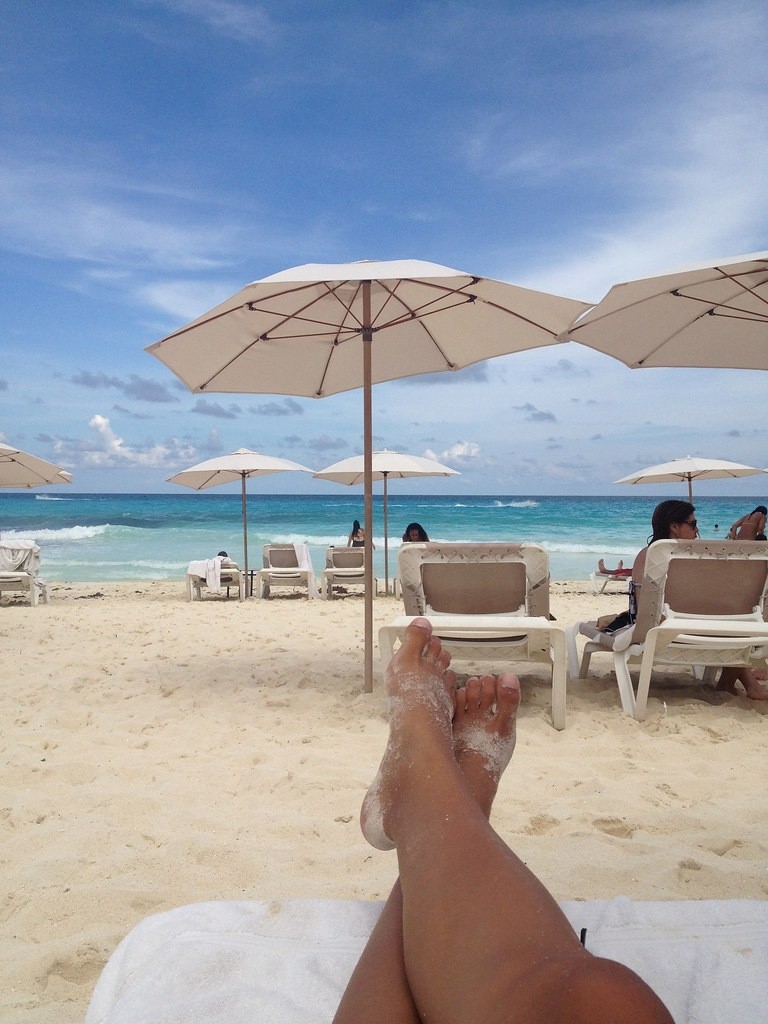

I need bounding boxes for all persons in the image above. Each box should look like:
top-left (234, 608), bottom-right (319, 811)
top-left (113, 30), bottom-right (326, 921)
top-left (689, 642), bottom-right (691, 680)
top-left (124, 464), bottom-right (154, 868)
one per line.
top-left (347, 520), bottom-right (376, 550)
top-left (218, 551), bottom-right (227, 557)
top-left (332, 616), bottom-right (675, 1024)
top-left (605, 500), bottom-right (699, 630)
top-left (598, 559), bottom-right (633, 576)
top-left (724, 505), bottom-right (768, 540)
top-left (403, 523), bottom-right (429, 541)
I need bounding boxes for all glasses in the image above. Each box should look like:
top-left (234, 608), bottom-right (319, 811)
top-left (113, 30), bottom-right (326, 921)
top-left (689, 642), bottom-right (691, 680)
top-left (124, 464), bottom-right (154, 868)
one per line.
top-left (670, 520), bottom-right (696, 527)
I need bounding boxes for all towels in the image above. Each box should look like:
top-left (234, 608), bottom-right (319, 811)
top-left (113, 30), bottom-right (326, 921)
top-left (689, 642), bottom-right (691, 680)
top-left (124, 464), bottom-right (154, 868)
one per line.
top-left (0, 539), bottom-right (48, 588)
top-left (292, 542), bottom-right (321, 601)
top-left (188, 556), bottom-right (233, 592)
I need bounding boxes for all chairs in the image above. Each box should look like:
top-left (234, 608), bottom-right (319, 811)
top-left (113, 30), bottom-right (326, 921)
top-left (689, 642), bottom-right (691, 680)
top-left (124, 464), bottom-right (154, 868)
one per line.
top-left (378, 542), bottom-right (567, 730)
top-left (591, 571), bottom-right (631, 593)
top-left (578, 540), bottom-right (768, 721)
top-left (258, 544), bottom-right (315, 600)
top-left (188, 560), bottom-right (250, 601)
top-left (0, 540), bottom-right (50, 605)
top-left (321, 547), bottom-right (377, 600)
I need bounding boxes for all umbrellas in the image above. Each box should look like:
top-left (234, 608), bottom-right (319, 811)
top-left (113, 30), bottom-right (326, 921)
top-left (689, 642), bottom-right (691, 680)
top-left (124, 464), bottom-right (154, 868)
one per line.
top-left (166, 446), bottom-right (316, 599)
top-left (612, 455), bottom-right (764, 504)
top-left (141, 259), bottom-right (596, 694)
top-left (0, 443), bottom-right (72, 488)
top-left (314, 448), bottom-right (462, 596)
top-left (555, 251), bottom-right (768, 370)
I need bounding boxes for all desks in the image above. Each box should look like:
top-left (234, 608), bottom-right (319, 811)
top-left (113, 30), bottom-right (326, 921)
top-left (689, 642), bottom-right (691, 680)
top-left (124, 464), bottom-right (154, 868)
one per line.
top-left (227, 569), bottom-right (260, 597)
